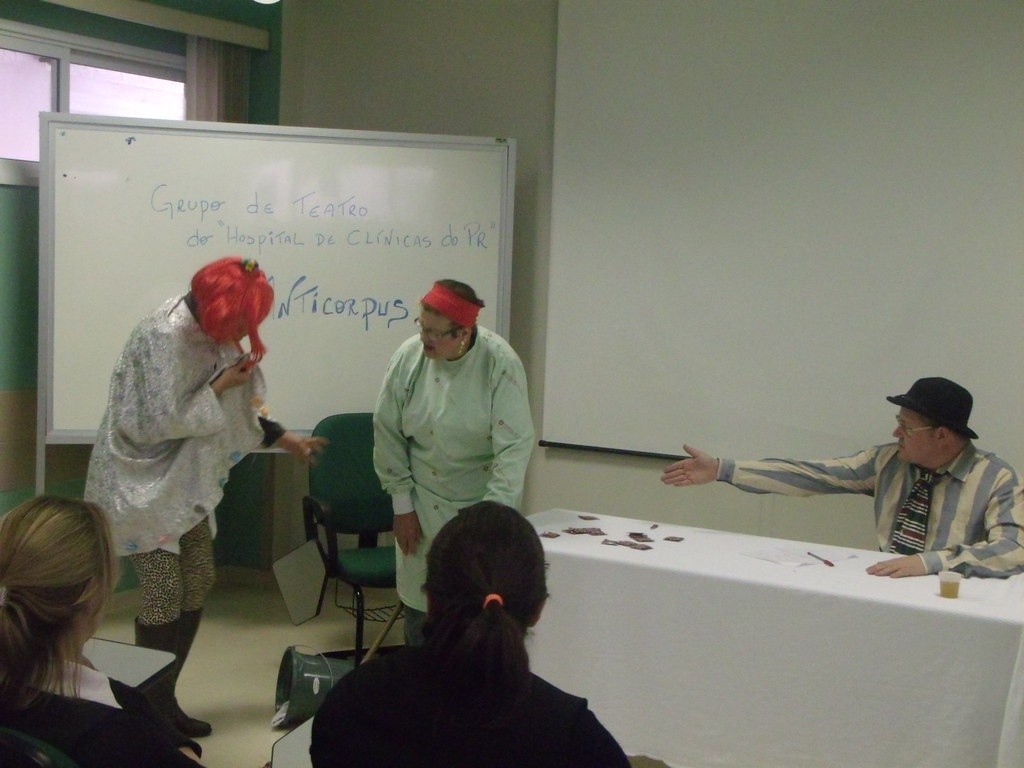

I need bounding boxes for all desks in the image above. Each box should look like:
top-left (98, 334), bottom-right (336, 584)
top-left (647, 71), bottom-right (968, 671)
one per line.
top-left (524, 509), bottom-right (1024, 768)
top-left (82, 636), bottom-right (178, 692)
top-left (272, 716), bottom-right (317, 768)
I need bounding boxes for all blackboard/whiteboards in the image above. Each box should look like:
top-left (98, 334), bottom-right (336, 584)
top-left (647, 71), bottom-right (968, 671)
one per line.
top-left (37, 110), bottom-right (517, 444)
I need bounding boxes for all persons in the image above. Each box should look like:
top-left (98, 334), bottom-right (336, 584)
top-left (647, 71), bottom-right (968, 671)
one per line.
top-left (661, 376), bottom-right (1024, 578)
top-left (311, 500), bottom-right (632, 768)
top-left (86, 256), bottom-right (330, 737)
top-left (373, 280), bottom-right (534, 646)
top-left (0, 496), bottom-right (205, 768)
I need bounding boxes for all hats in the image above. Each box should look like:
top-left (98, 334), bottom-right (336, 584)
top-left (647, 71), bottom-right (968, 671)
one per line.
top-left (887, 377), bottom-right (978, 439)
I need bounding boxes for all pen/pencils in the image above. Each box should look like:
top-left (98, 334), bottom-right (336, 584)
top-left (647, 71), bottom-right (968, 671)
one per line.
top-left (807, 551), bottom-right (834, 567)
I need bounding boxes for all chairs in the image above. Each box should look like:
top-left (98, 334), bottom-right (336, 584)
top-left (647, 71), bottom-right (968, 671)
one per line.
top-left (302, 413), bottom-right (401, 666)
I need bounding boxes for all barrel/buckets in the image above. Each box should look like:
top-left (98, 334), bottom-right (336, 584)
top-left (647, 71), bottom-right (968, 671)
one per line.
top-left (275, 644), bottom-right (356, 725)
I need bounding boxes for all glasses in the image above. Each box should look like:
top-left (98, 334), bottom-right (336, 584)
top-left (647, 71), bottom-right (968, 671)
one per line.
top-left (414, 317), bottom-right (462, 341)
top-left (896, 415), bottom-right (939, 438)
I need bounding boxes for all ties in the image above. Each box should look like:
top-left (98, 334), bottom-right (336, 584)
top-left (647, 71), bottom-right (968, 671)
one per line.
top-left (889, 464), bottom-right (952, 555)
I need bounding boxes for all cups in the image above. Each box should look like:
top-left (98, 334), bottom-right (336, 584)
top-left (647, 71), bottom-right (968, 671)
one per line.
top-left (939, 571), bottom-right (961, 597)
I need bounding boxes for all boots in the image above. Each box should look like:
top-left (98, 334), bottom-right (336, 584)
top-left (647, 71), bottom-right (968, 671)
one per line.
top-left (134, 615), bottom-right (183, 733)
top-left (171, 607), bottom-right (212, 737)
